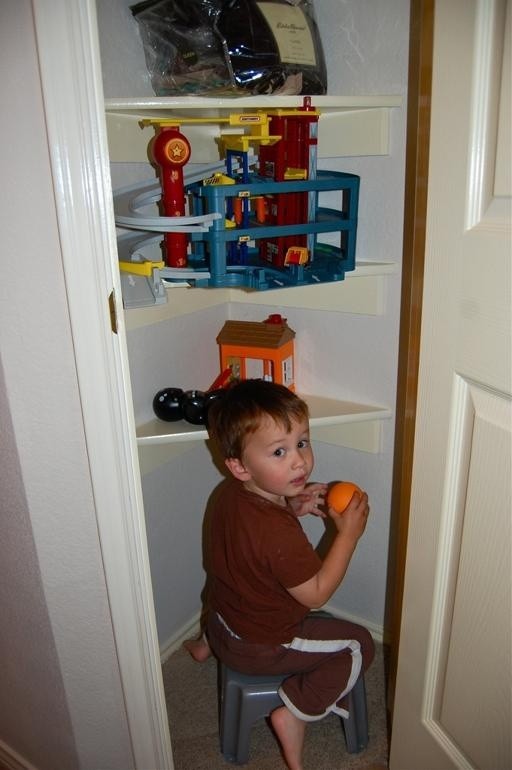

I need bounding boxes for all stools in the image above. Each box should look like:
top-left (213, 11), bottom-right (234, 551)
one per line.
top-left (217, 642), bottom-right (372, 768)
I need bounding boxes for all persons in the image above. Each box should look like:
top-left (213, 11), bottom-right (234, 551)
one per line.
top-left (182, 379), bottom-right (375, 769)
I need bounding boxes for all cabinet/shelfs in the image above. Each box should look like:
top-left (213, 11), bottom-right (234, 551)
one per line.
top-left (87, 0), bottom-right (403, 662)
